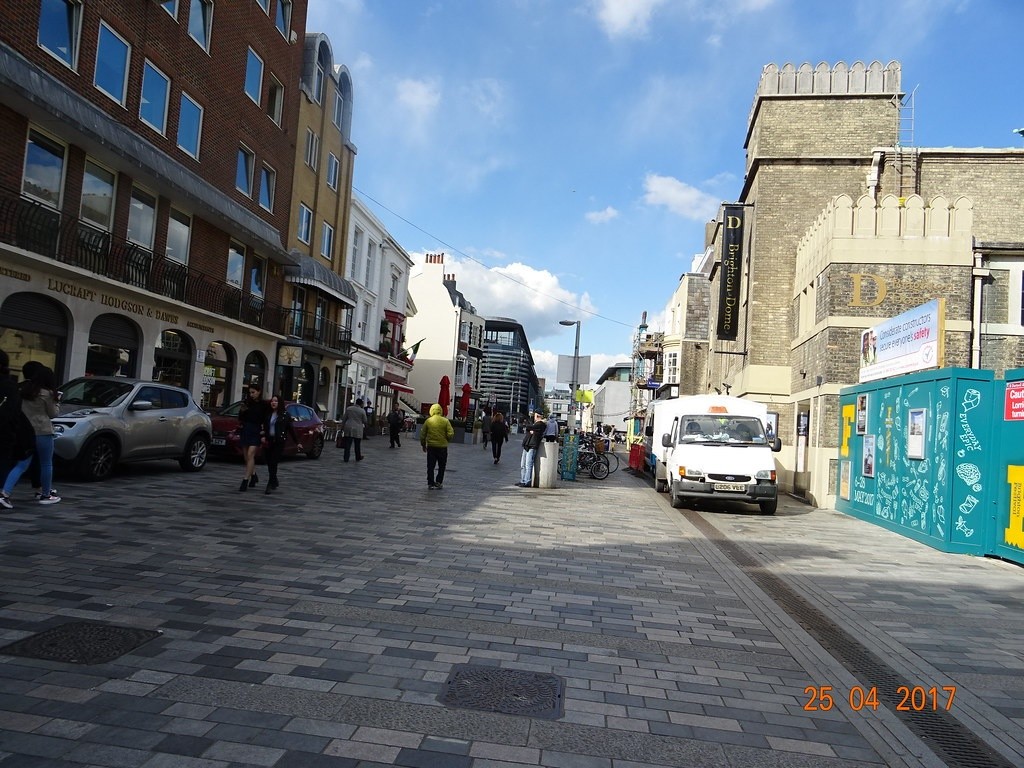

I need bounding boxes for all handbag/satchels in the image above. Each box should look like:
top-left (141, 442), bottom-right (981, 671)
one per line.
top-left (335, 431), bottom-right (344, 448)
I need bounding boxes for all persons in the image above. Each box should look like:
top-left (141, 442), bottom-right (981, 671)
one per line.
top-left (0, 348), bottom-right (62, 509)
top-left (238, 384), bottom-right (303, 494)
top-left (482, 414), bottom-right (492, 450)
top-left (491, 413), bottom-right (508, 464)
top-left (608, 425), bottom-right (626, 444)
top-left (420, 403), bottom-right (454, 489)
top-left (339, 399), bottom-right (371, 462)
top-left (862, 330), bottom-right (877, 367)
top-left (543, 415), bottom-right (559, 443)
top-left (387, 402), bottom-right (404, 448)
top-left (515, 408), bottom-right (547, 488)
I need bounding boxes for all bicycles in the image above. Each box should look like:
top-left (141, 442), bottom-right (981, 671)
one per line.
top-left (557, 430), bottom-right (619, 481)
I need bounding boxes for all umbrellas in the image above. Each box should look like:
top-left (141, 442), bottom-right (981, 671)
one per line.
top-left (438, 375), bottom-right (450, 418)
top-left (460, 383), bottom-right (471, 421)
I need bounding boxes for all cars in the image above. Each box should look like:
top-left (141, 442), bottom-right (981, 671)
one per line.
top-left (50, 375), bottom-right (213, 480)
top-left (208, 396), bottom-right (325, 466)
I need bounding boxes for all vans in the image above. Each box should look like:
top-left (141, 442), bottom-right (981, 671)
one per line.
top-left (643, 394), bottom-right (783, 516)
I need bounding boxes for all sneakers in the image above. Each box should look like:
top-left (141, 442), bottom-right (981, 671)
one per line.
top-left (0, 493), bottom-right (13, 508)
top-left (39, 494), bottom-right (61, 504)
top-left (33, 488), bottom-right (57, 499)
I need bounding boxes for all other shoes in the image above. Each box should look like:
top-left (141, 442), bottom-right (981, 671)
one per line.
top-left (343, 457), bottom-right (349, 461)
top-left (493, 458), bottom-right (499, 464)
top-left (389, 446), bottom-right (395, 448)
top-left (265, 479), bottom-right (279, 494)
top-left (428, 486), bottom-right (434, 489)
top-left (515, 482), bottom-right (531, 487)
top-left (397, 444), bottom-right (401, 447)
top-left (435, 482), bottom-right (443, 487)
top-left (356, 456), bottom-right (364, 460)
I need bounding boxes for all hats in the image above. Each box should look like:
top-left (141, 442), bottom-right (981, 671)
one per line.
top-left (534, 409), bottom-right (544, 414)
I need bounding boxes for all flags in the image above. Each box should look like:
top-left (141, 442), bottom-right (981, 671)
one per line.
top-left (409, 339), bottom-right (425, 362)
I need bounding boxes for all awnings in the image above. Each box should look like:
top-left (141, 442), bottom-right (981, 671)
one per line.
top-left (627, 408), bottom-right (647, 419)
top-left (378, 375), bottom-right (415, 395)
top-left (284, 253), bottom-right (358, 309)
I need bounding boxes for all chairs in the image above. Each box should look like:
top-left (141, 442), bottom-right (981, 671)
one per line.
top-left (321, 418), bottom-right (345, 442)
top-left (685, 421), bottom-right (751, 440)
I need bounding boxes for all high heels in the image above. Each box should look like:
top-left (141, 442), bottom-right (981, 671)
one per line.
top-left (249, 472), bottom-right (258, 487)
top-left (239, 478), bottom-right (249, 492)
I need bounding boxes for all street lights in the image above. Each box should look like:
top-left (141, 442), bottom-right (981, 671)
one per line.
top-left (559, 319), bottom-right (581, 435)
top-left (510, 381), bottom-right (522, 424)
